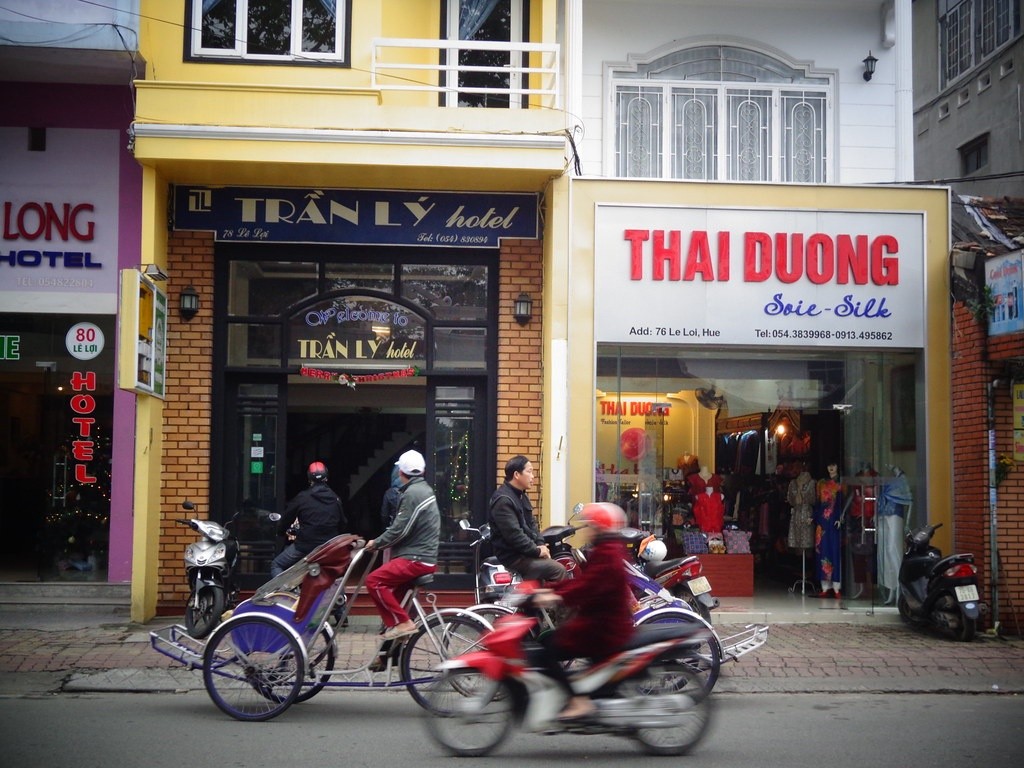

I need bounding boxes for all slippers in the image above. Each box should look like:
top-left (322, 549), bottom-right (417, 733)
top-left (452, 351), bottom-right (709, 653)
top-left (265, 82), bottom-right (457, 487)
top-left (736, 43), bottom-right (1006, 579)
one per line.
top-left (370, 655), bottom-right (387, 670)
top-left (379, 620), bottom-right (419, 640)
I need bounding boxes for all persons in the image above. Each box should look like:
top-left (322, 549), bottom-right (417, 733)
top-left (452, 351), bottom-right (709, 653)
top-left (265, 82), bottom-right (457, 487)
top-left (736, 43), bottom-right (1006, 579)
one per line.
top-left (688, 467), bottom-right (723, 500)
top-left (787, 472), bottom-right (816, 549)
top-left (879, 464), bottom-right (913, 606)
top-left (534, 503), bottom-right (634, 718)
top-left (270, 462), bottom-right (345, 580)
top-left (365, 450), bottom-right (441, 671)
top-left (381, 465), bottom-right (405, 526)
top-left (813, 460), bottom-right (848, 598)
top-left (488, 456), bottom-right (568, 581)
top-left (695, 486), bottom-right (725, 532)
top-left (840, 463), bottom-right (881, 599)
top-left (678, 449), bottom-right (700, 477)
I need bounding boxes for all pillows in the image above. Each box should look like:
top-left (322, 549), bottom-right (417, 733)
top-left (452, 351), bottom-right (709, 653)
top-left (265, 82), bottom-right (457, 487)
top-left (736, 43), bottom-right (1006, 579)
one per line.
top-left (680, 531), bottom-right (709, 555)
top-left (722, 529), bottom-right (753, 554)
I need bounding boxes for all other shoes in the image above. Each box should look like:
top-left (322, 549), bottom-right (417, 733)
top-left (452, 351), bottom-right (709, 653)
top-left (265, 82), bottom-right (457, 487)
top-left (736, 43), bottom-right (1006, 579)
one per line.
top-left (337, 618), bottom-right (349, 629)
top-left (557, 695), bottom-right (596, 720)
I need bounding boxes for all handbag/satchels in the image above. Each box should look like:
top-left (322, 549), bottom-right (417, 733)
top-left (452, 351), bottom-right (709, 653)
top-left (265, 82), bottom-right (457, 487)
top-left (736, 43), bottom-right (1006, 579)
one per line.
top-left (723, 528), bottom-right (750, 553)
top-left (681, 531), bottom-right (709, 555)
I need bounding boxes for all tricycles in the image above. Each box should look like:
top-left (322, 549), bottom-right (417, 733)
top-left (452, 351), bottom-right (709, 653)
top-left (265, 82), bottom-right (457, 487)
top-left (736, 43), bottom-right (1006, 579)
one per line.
top-left (148, 531), bottom-right (495, 723)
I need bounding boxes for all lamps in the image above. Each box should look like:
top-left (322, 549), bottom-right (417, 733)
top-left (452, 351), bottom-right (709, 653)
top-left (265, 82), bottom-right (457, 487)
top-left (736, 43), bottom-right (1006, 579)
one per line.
top-left (178, 284), bottom-right (200, 321)
top-left (512, 292), bottom-right (533, 326)
top-left (862, 50), bottom-right (879, 81)
top-left (139, 263), bottom-right (169, 281)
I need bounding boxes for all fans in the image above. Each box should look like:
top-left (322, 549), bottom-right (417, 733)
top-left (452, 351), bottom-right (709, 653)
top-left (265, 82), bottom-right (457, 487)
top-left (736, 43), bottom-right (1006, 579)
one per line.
top-left (694, 383), bottom-right (723, 410)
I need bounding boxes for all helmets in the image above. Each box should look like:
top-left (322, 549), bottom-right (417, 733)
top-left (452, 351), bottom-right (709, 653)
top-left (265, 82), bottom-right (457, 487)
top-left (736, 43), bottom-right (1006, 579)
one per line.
top-left (307, 461), bottom-right (326, 481)
top-left (640, 540), bottom-right (666, 563)
top-left (580, 501), bottom-right (628, 536)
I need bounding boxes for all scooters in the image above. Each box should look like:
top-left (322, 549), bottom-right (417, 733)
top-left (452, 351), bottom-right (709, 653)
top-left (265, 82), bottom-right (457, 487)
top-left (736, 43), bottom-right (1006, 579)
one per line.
top-left (429, 578), bottom-right (718, 759)
top-left (897, 519), bottom-right (987, 644)
top-left (168, 500), bottom-right (247, 640)
top-left (471, 500), bottom-right (721, 687)
top-left (265, 512), bottom-right (348, 630)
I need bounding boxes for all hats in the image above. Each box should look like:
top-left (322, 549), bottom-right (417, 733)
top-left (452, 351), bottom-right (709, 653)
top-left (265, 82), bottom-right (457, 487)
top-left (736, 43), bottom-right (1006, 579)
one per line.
top-left (394, 449), bottom-right (425, 478)
